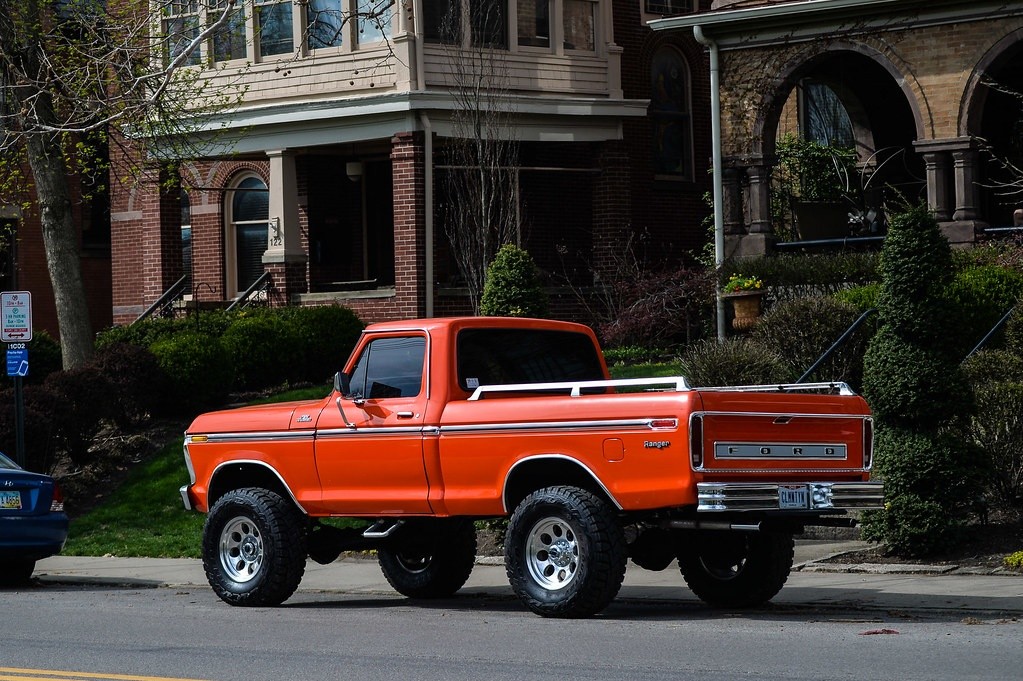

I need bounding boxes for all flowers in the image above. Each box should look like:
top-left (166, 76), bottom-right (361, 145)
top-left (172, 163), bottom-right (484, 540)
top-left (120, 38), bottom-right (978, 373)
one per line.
top-left (724, 272), bottom-right (765, 293)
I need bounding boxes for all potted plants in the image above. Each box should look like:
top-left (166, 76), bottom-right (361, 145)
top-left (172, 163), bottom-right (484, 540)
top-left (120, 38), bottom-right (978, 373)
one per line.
top-left (777, 129), bottom-right (856, 256)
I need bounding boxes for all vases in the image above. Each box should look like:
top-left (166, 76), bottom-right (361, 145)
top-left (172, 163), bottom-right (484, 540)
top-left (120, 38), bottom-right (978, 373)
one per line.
top-left (720, 288), bottom-right (767, 337)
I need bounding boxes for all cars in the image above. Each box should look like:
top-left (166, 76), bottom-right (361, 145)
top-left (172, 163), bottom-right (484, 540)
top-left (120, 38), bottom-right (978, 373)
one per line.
top-left (0, 450), bottom-right (69, 585)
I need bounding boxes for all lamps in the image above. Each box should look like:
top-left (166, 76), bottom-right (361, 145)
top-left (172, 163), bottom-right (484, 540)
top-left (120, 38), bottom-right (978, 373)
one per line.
top-left (346, 142), bottom-right (364, 181)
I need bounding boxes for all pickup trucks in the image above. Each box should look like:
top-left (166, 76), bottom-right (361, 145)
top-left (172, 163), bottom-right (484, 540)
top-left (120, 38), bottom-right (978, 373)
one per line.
top-left (178, 316), bottom-right (879, 619)
